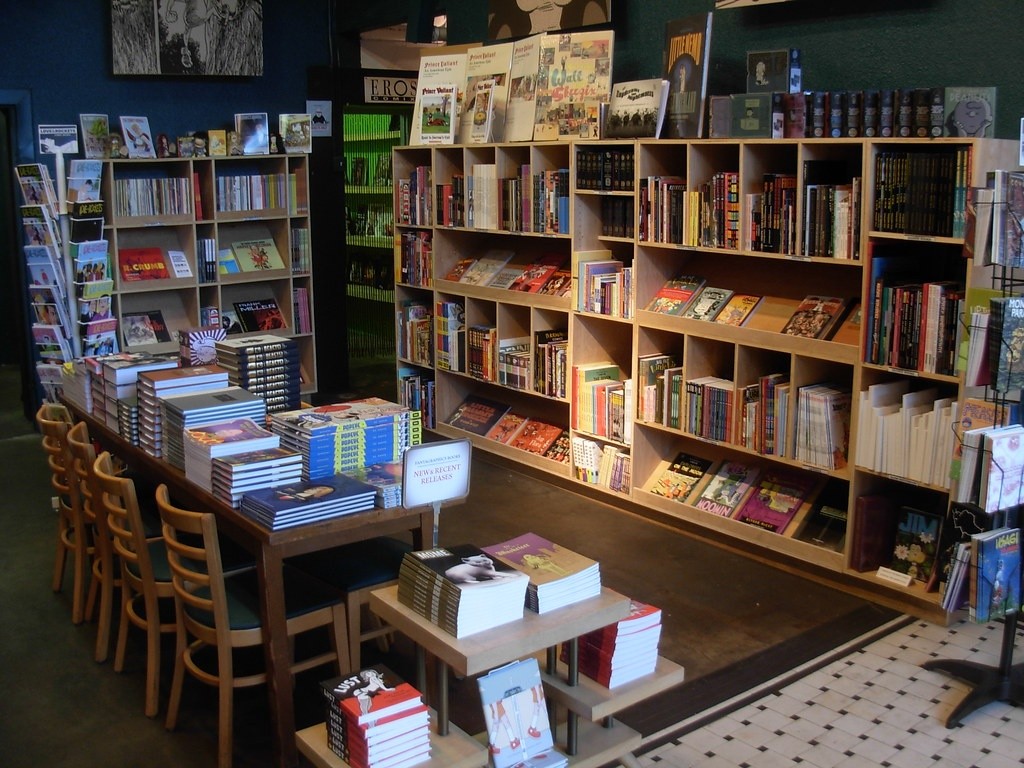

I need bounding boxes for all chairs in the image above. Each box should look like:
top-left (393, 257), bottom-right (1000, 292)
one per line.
top-left (37, 401), bottom-right (139, 593)
top-left (153, 484), bottom-right (351, 768)
top-left (92, 450), bottom-right (199, 719)
top-left (286, 536), bottom-right (416, 673)
top-left (65, 420), bottom-right (160, 663)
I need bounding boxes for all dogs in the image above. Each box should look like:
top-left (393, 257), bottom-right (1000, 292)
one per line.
top-left (444, 553), bottom-right (518, 584)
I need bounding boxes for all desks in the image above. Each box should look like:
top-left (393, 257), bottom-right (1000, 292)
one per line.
top-left (54, 385), bottom-right (467, 768)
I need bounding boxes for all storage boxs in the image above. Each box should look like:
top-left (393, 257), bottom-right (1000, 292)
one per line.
top-left (746, 47), bottom-right (803, 95)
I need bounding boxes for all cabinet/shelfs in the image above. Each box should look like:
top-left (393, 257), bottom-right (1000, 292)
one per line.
top-left (295, 585), bottom-right (684, 768)
top-left (381, 138), bottom-right (1024, 627)
top-left (343, 113), bottom-right (395, 304)
top-left (101, 154), bottom-right (318, 405)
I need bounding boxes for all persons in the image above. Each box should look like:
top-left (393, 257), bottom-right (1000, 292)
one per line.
top-left (653, 108), bottom-right (658, 122)
top-left (608, 110), bottom-right (613, 122)
top-left (492, 74), bottom-right (501, 86)
top-left (613, 110), bottom-right (622, 138)
top-left (631, 110), bottom-right (642, 139)
top-left (623, 112), bottom-right (630, 138)
top-left (643, 109), bottom-right (648, 116)
top-left (643, 114), bottom-right (656, 137)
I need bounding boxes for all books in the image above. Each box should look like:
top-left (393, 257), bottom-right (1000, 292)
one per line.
top-left (396, 148), bottom-right (1024, 692)
top-left (15, 112), bottom-right (422, 531)
top-left (321, 658), bottom-right (568, 768)
top-left (408, 11), bottom-right (997, 145)
